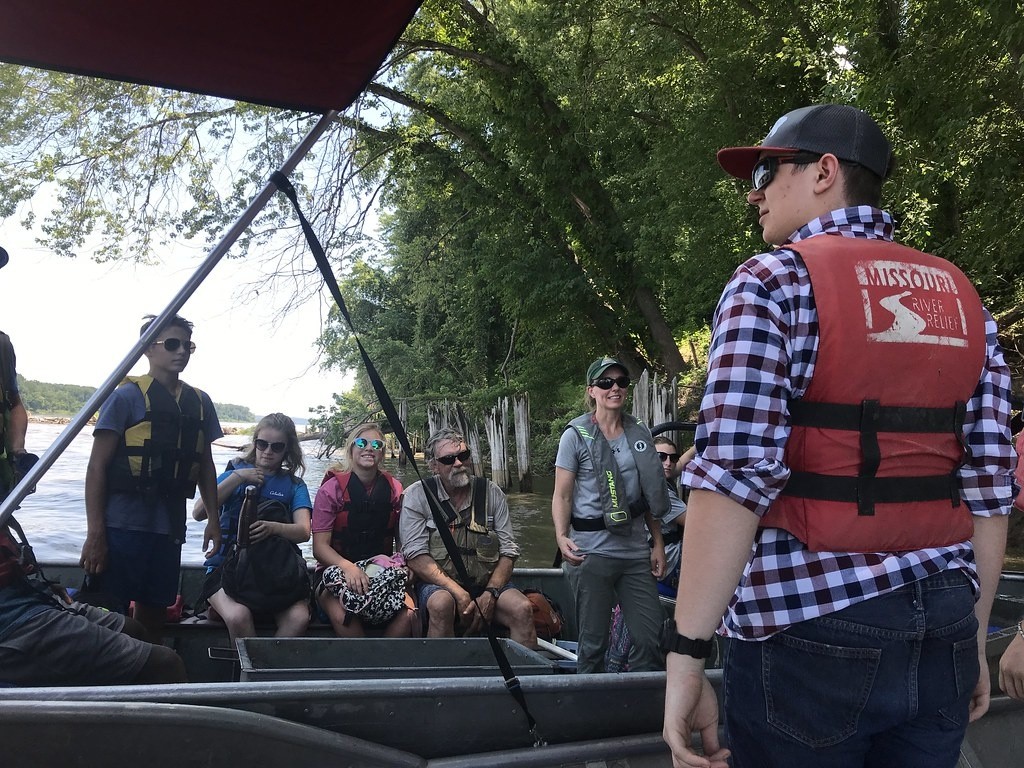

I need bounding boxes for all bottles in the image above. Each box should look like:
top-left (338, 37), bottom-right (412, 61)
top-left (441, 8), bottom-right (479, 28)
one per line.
top-left (236, 485), bottom-right (257, 549)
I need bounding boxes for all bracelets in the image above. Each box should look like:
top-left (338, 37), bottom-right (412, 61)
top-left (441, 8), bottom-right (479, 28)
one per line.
top-left (1018, 621), bottom-right (1024, 639)
top-left (233, 470), bottom-right (246, 483)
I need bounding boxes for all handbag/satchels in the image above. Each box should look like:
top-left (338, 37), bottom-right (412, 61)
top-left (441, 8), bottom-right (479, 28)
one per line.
top-left (323, 555), bottom-right (406, 625)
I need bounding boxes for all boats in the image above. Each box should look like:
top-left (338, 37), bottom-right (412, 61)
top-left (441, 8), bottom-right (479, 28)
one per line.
top-left (0, 0), bottom-right (1024, 768)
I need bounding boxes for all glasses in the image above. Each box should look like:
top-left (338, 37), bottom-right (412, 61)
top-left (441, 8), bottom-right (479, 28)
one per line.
top-left (657, 452), bottom-right (680, 463)
top-left (355, 438), bottom-right (386, 450)
top-left (255, 439), bottom-right (288, 453)
top-left (152, 338), bottom-right (196, 354)
top-left (751, 155), bottom-right (857, 191)
top-left (433, 446), bottom-right (471, 465)
top-left (590, 376), bottom-right (631, 390)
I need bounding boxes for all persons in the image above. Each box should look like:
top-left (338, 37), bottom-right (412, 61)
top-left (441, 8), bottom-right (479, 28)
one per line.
top-left (79, 314), bottom-right (224, 644)
top-left (0, 330), bottom-right (39, 523)
top-left (0, 514), bottom-right (186, 687)
top-left (399, 428), bottom-right (537, 651)
top-left (192, 413), bottom-right (312, 649)
top-left (654, 436), bottom-right (697, 582)
top-left (312, 423), bottom-right (418, 639)
top-left (997, 423), bottom-right (1024, 701)
top-left (657, 104), bottom-right (1021, 768)
top-left (552, 357), bottom-right (672, 674)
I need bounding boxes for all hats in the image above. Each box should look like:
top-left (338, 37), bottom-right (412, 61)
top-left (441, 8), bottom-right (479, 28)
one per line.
top-left (586, 358), bottom-right (630, 386)
top-left (717, 104), bottom-right (892, 180)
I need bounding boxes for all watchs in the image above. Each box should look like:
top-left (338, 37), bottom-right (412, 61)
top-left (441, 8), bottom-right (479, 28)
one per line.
top-left (658, 618), bottom-right (713, 659)
top-left (485, 587), bottom-right (500, 598)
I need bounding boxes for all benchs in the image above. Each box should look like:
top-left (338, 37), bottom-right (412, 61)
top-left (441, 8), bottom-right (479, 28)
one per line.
top-left (163, 608), bottom-right (511, 682)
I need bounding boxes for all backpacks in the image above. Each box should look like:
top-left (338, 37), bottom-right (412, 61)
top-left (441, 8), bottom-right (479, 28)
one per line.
top-left (222, 499), bottom-right (313, 606)
